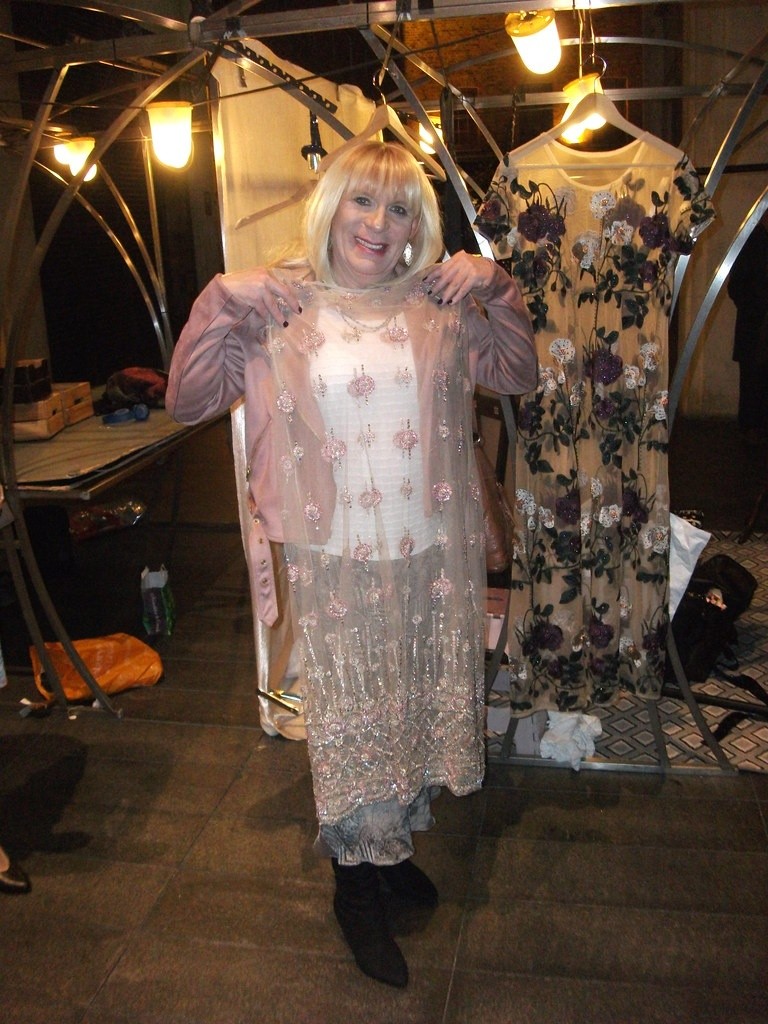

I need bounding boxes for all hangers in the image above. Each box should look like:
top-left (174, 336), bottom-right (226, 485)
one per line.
top-left (501, 53), bottom-right (689, 170)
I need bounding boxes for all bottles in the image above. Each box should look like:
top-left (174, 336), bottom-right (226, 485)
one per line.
top-left (140, 557), bottom-right (178, 634)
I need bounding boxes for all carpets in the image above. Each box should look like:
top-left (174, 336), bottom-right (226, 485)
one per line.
top-left (488, 529), bottom-right (768, 775)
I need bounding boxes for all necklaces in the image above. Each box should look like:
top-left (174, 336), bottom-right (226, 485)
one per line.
top-left (321, 278), bottom-right (401, 331)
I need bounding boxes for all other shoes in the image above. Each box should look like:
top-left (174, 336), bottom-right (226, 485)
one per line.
top-left (0, 846), bottom-right (31, 892)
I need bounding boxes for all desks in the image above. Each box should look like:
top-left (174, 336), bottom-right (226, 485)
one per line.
top-left (0, 383), bottom-right (219, 716)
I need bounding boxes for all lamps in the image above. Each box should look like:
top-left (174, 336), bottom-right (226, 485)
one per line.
top-left (52, 124), bottom-right (98, 182)
top-left (143, 81), bottom-right (194, 170)
top-left (504, 8), bottom-right (562, 75)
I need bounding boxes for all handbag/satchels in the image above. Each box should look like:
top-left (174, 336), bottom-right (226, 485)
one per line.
top-left (661, 551), bottom-right (757, 681)
top-left (139, 563), bottom-right (176, 636)
top-left (31, 632), bottom-right (162, 702)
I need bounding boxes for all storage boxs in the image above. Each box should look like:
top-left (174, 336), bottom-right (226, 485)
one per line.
top-left (0, 357), bottom-right (52, 402)
top-left (52, 382), bottom-right (94, 425)
top-left (12, 392), bottom-right (64, 441)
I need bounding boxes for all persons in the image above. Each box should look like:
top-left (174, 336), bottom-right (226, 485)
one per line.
top-left (167, 141), bottom-right (536, 990)
top-left (0, 845), bottom-right (31, 893)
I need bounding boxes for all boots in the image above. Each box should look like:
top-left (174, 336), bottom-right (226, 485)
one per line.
top-left (331, 857), bottom-right (409, 989)
top-left (379, 854), bottom-right (439, 909)
